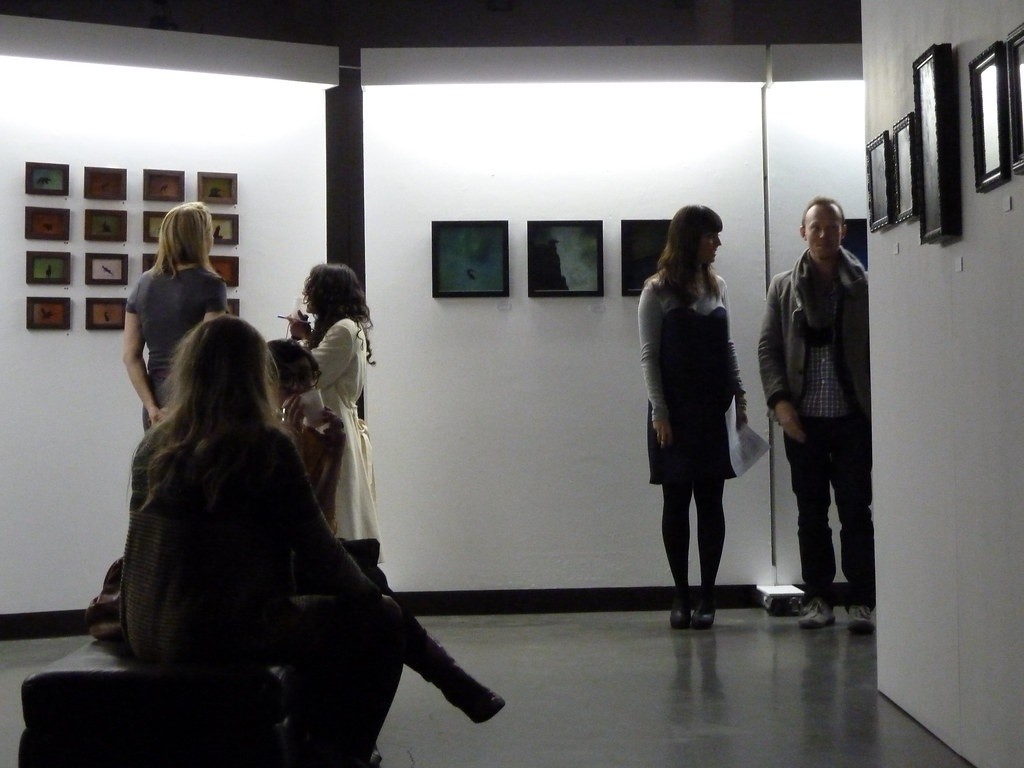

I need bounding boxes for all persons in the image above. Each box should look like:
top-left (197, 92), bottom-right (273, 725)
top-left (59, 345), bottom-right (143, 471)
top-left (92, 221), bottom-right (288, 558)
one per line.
top-left (759, 197), bottom-right (876, 632)
top-left (268, 342), bottom-right (346, 532)
top-left (637, 203), bottom-right (748, 629)
top-left (289, 263), bottom-right (385, 565)
top-left (122, 201), bottom-right (228, 431)
top-left (120, 317), bottom-right (506, 768)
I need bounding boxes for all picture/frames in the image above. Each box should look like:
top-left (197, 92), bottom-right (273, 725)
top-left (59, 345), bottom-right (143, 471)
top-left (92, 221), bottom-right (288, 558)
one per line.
top-left (84, 167), bottom-right (127, 201)
top-left (143, 211), bottom-right (168, 243)
top-left (143, 169), bottom-right (185, 202)
top-left (85, 298), bottom-right (127, 329)
top-left (227, 299), bottom-right (239, 317)
top-left (85, 209), bottom-right (128, 241)
top-left (527, 220), bottom-right (604, 297)
top-left (865, 130), bottom-right (893, 234)
top-left (621, 219), bottom-right (673, 296)
top-left (968, 41), bottom-right (1011, 193)
top-left (27, 297), bottom-right (70, 330)
top-left (912, 42), bottom-right (961, 245)
top-left (25, 162), bottom-right (69, 196)
top-left (197, 172), bottom-right (237, 204)
top-left (24, 206), bottom-right (70, 240)
top-left (142, 253), bottom-right (180, 272)
top-left (85, 253), bottom-right (128, 286)
top-left (892, 111), bottom-right (920, 224)
top-left (26, 251), bottom-right (70, 286)
top-left (209, 213), bottom-right (239, 245)
top-left (208, 256), bottom-right (239, 287)
top-left (431, 220), bottom-right (510, 298)
top-left (1005, 21), bottom-right (1024, 175)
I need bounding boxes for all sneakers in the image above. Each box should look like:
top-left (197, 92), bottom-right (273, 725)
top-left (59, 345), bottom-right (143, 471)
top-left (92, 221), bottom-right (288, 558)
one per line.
top-left (848, 604), bottom-right (874, 634)
top-left (799, 597), bottom-right (835, 628)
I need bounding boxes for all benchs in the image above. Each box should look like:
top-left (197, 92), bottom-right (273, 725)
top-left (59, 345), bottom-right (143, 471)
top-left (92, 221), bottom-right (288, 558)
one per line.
top-left (19, 640), bottom-right (292, 768)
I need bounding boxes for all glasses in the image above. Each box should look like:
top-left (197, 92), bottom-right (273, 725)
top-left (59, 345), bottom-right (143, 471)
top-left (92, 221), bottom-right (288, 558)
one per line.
top-left (278, 368), bottom-right (322, 390)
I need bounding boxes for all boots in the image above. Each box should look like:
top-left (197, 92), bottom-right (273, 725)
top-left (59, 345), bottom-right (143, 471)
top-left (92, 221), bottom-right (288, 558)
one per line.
top-left (354, 745), bottom-right (382, 768)
top-left (405, 628), bottom-right (505, 724)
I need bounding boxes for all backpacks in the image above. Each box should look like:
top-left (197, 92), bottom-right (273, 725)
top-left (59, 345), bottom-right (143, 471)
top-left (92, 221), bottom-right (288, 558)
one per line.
top-left (85, 555), bottom-right (124, 641)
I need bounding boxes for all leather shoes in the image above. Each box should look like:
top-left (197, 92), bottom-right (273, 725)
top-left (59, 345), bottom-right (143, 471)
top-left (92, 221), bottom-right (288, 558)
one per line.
top-left (692, 598), bottom-right (715, 628)
top-left (670, 594), bottom-right (693, 628)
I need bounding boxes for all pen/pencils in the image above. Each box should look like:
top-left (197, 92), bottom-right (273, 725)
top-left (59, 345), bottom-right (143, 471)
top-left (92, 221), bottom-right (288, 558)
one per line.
top-left (277, 315), bottom-right (311, 324)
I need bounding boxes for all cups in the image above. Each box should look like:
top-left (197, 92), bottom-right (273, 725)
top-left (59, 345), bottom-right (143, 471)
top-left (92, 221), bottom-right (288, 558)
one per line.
top-left (298, 389), bottom-right (328, 429)
